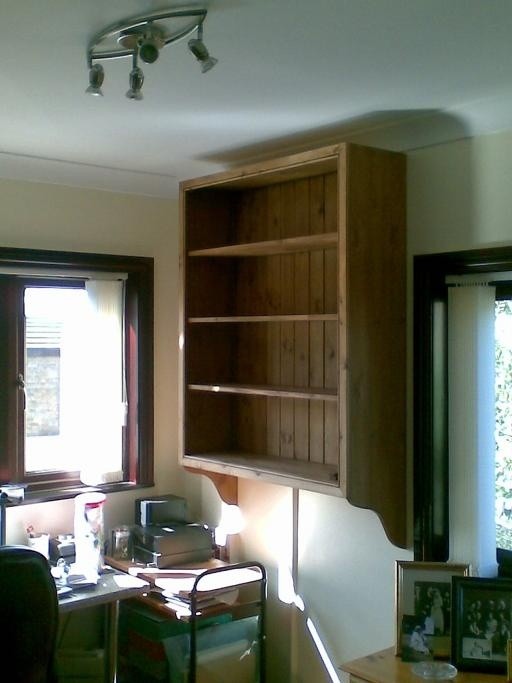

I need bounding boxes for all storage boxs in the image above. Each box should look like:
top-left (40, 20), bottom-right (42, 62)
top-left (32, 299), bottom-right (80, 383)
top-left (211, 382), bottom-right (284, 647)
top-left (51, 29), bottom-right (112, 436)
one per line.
top-left (127, 526), bottom-right (217, 565)
top-left (128, 603), bottom-right (234, 681)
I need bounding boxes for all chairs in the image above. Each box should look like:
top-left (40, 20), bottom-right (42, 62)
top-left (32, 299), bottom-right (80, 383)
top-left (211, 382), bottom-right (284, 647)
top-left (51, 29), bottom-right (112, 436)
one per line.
top-left (0, 544), bottom-right (59, 681)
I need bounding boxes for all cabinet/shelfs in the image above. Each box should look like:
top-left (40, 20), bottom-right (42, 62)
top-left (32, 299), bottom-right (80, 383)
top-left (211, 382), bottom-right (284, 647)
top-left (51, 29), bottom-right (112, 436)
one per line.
top-left (178, 140), bottom-right (406, 550)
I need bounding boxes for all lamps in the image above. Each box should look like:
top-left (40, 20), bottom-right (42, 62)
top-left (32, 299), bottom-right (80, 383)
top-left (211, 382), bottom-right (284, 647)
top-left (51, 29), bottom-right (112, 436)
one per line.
top-left (85, 7), bottom-right (219, 102)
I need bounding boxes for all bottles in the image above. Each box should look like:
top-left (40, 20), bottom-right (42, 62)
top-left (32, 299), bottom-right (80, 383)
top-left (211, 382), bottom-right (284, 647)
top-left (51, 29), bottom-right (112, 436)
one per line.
top-left (115, 526), bottom-right (131, 558)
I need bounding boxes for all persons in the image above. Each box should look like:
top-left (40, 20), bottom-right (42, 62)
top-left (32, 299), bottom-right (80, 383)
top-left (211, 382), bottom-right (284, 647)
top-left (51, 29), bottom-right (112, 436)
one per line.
top-left (400, 581), bottom-right (511, 662)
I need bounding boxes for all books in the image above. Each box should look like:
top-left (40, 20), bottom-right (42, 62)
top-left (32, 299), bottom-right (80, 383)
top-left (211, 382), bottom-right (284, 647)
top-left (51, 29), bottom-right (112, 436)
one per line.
top-left (43, 558), bottom-right (229, 618)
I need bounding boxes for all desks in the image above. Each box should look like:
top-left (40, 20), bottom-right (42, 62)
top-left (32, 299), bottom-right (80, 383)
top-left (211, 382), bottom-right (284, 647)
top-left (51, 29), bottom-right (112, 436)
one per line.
top-left (337, 644), bottom-right (511, 683)
top-left (105, 553), bottom-right (267, 682)
top-left (49, 559), bottom-right (151, 683)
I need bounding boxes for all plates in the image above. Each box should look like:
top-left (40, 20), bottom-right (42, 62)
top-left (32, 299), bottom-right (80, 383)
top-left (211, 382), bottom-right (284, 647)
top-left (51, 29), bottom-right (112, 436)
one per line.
top-left (411, 659), bottom-right (459, 680)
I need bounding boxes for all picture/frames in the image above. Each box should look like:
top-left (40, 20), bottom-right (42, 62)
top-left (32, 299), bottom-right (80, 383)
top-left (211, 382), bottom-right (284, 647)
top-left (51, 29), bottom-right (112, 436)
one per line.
top-left (451, 575), bottom-right (511, 673)
top-left (396, 560), bottom-right (470, 659)
